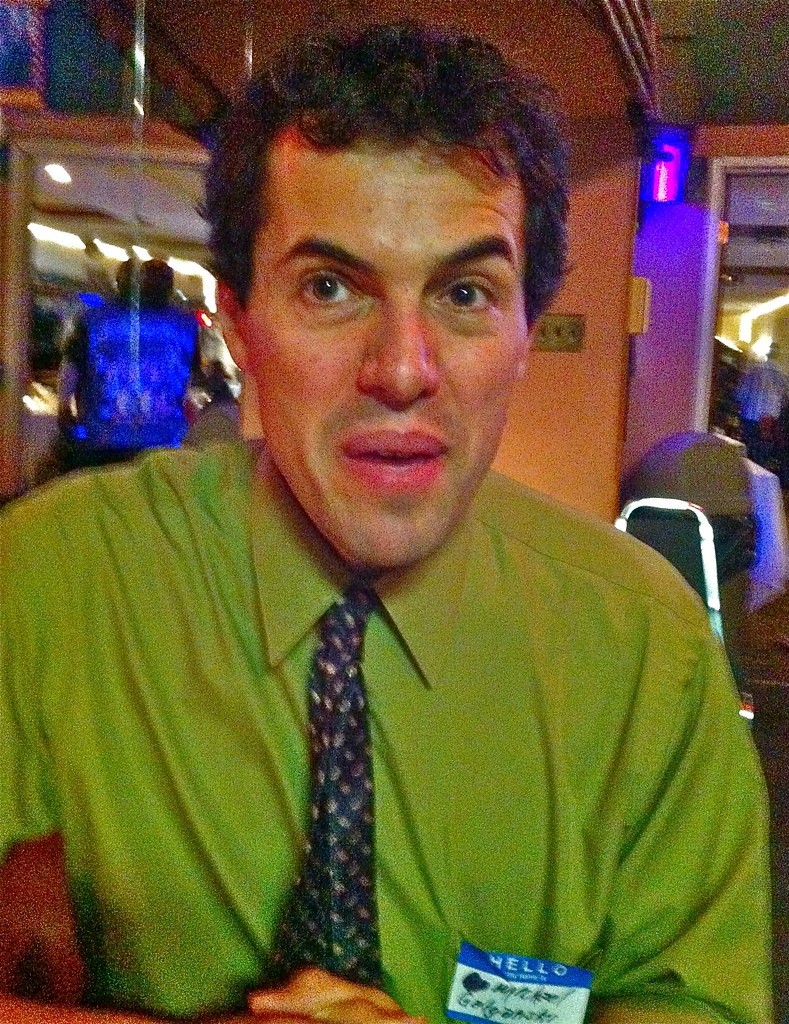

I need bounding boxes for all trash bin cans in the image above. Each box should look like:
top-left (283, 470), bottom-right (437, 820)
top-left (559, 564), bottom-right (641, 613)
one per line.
top-left (616, 422), bottom-right (762, 693)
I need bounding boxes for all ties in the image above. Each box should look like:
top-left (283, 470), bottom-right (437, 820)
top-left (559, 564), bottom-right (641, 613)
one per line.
top-left (273, 580), bottom-right (384, 993)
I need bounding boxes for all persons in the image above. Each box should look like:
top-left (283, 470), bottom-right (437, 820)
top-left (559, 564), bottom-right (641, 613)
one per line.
top-left (0, 23), bottom-right (772, 1023)
top-left (734, 342), bottom-right (788, 467)
top-left (57, 258), bottom-right (203, 471)
